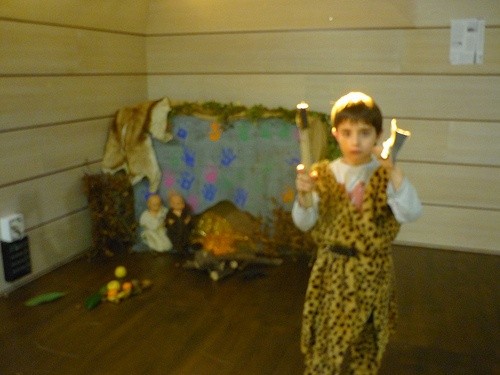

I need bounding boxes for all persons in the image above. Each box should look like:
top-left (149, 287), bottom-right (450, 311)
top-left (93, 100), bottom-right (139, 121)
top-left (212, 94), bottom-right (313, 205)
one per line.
top-left (166, 194), bottom-right (199, 256)
top-left (137, 188), bottom-right (175, 254)
top-left (289, 93), bottom-right (423, 373)
